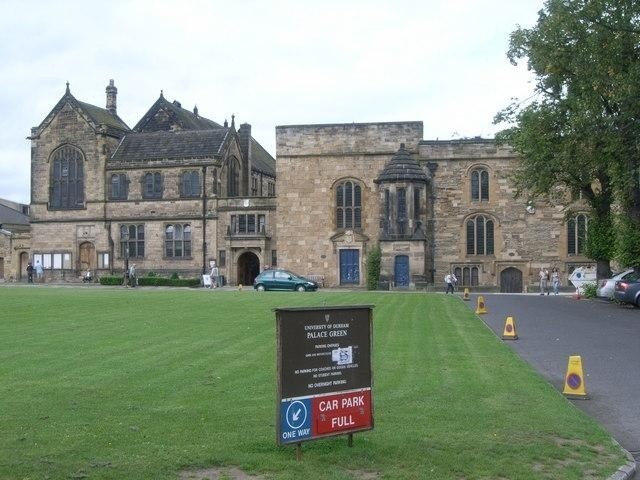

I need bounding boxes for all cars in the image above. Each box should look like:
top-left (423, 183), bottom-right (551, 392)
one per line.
top-left (614, 269), bottom-right (640, 309)
top-left (253, 269), bottom-right (318, 292)
top-left (596, 266), bottom-right (638, 306)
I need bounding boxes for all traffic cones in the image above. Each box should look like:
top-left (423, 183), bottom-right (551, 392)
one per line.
top-left (476, 297), bottom-right (488, 314)
top-left (462, 288), bottom-right (471, 301)
top-left (562, 355), bottom-right (592, 401)
top-left (501, 315), bottom-right (518, 341)
top-left (573, 286), bottom-right (582, 298)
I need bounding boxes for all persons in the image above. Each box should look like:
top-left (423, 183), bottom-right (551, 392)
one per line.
top-left (82, 269), bottom-right (93, 284)
top-left (122, 264), bottom-right (130, 287)
top-left (35, 261), bottom-right (44, 280)
top-left (538, 266), bottom-right (550, 295)
top-left (26, 261), bottom-right (35, 283)
top-left (208, 264), bottom-right (218, 289)
top-left (129, 264), bottom-right (137, 287)
top-left (550, 267), bottom-right (560, 296)
top-left (445, 271), bottom-right (458, 294)
